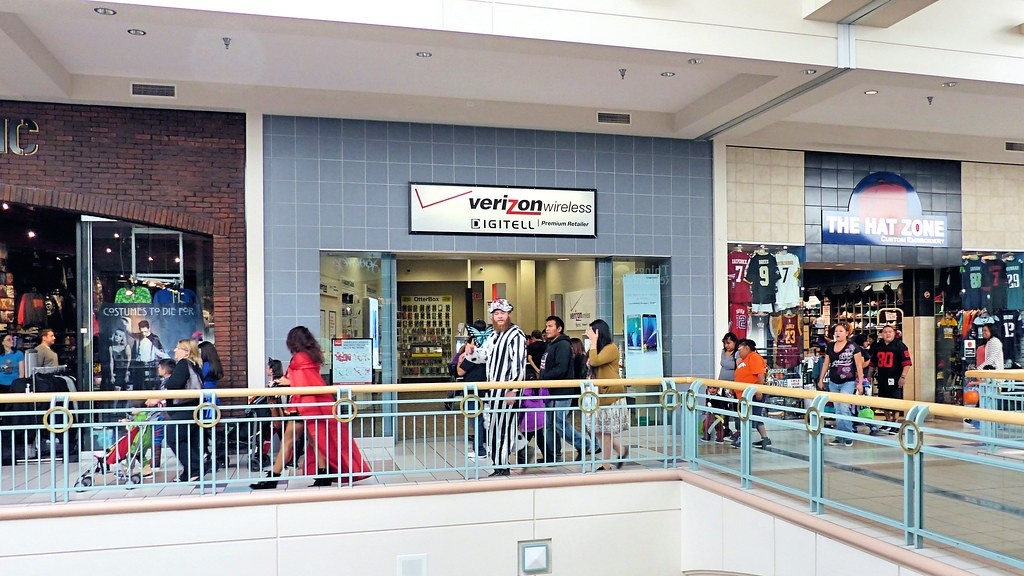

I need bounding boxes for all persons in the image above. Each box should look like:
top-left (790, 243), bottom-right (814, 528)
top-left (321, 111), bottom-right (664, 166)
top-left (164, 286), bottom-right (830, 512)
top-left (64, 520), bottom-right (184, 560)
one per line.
top-left (257, 357), bottom-right (300, 470)
top-left (109, 317), bottom-right (172, 414)
top-left (818, 322), bottom-right (912, 447)
top-left (16, 286), bottom-right (67, 331)
top-left (0, 329), bottom-right (59, 443)
top-left (963, 323), bottom-right (1005, 430)
top-left (452, 298), bottom-right (629, 477)
top-left (114, 339), bottom-right (230, 482)
top-left (700, 332), bottom-right (772, 448)
top-left (249, 326), bottom-right (373, 490)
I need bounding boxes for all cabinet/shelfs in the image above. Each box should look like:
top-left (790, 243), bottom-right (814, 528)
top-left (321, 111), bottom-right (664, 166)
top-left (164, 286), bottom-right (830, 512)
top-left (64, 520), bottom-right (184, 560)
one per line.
top-left (805, 289), bottom-right (900, 347)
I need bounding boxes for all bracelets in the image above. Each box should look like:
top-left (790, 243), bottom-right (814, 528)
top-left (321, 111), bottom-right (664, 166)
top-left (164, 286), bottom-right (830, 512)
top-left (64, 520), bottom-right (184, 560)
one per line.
top-left (590, 343), bottom-right (597, 346)
top-left (901, 376), bottom-right (905, 380)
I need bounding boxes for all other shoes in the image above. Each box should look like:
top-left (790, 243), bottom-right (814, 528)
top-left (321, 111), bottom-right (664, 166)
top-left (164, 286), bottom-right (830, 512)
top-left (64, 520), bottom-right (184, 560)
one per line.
top-left (113, 472), bottom-right (129, 479)
top-left (467, 451), bottom-right (487, 459)
top-left (596, 446), bottom-right (629, 470)
top-left (143, 459), bottom-right (160, 479)
top-left (517, 444), bottom-right (535, 468)
top-left (488, 468), bottom-right (510, 477)
top-left (536, 456), bottom-right (566, 467)
top-left (173, 452), bottom-right (230, 482)
top-left (575, 445), bottom-right (602, 461)
top-left (964, 419), bottom-right (980, 427)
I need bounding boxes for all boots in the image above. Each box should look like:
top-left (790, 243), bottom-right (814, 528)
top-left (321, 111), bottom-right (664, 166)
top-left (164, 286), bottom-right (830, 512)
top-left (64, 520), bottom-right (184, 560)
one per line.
top-left (307, 468), bottom-right (332, 487)
top-left (248, 470), bottom-right (282, 490)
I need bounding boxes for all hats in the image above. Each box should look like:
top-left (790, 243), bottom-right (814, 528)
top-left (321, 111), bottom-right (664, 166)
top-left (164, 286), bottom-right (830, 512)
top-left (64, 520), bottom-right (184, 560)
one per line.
top-left (489, 298), bottom-right (514, 314)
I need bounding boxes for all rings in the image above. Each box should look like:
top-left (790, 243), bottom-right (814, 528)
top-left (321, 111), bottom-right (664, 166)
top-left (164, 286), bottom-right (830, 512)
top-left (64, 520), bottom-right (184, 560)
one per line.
top-left (145, 401), bottom-right (148, 404)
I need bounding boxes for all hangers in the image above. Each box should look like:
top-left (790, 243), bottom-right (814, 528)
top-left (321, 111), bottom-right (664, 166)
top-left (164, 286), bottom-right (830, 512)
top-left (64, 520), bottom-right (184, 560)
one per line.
top-left (30, 365), bottom-right (66, 381)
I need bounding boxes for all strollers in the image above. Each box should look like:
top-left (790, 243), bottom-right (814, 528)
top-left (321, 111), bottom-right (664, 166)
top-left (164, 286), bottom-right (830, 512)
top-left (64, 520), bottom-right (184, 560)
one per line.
top-left (207, 383), bottom-right (278, 475)
top-left (73, 402), bottom-right (164, 492)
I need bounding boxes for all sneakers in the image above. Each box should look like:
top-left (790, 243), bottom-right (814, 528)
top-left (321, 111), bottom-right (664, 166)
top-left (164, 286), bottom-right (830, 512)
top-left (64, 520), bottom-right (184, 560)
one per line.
top-left (752, 437), bottom-right (771, 448)
top-left (868, 425), bottom-right (900, 436)
top-left (714, 428), bottom-right (742, 449)
top-left (828, 439), bottom-right (854, 447)
top-left (700, 437), bottom-right (711, 443)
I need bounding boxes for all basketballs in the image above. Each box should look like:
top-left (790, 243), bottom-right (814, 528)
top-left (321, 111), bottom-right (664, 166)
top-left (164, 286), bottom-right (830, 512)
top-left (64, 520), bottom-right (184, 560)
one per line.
top-left (963, 390), bottom-right (979, 405)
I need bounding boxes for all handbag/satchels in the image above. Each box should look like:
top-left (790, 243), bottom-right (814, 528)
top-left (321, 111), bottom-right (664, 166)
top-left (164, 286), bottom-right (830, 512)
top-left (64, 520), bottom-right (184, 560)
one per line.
top-left (173, 358), bottom-right (204, 406)
top-left (858, 408), bottom-right (874, 420)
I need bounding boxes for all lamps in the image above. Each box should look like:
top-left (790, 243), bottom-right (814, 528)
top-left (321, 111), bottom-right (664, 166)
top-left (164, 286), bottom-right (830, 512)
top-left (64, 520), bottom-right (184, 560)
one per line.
top-left (802, 281), bottom-right (895, 302)
